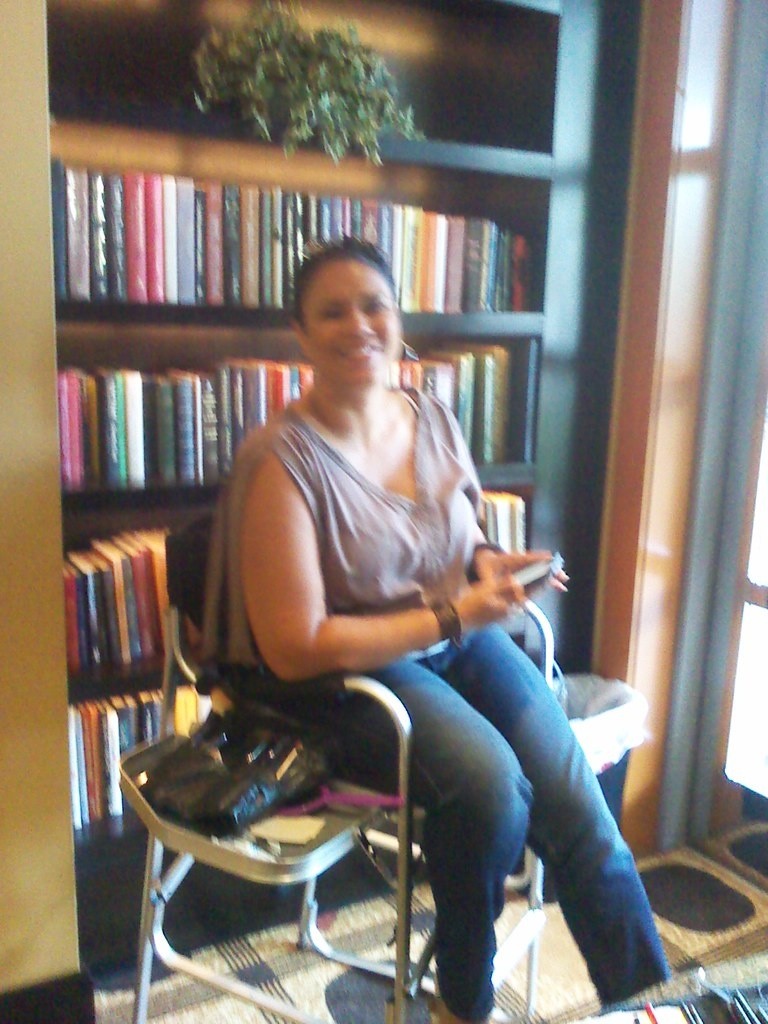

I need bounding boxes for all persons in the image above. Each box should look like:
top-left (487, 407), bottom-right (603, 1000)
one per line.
top-left (237, 239), bottom-right (675, 1024)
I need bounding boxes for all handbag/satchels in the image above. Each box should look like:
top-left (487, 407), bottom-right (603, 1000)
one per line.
top-left (140, 708), bottom-right (333, 838)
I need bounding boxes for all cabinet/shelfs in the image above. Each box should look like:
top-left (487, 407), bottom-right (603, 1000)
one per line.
top-left (50, 1), bottom-right (633, 954)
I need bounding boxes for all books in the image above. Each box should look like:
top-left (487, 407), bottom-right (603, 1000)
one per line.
top-left (300, 193), bottom-right (532, 311)
top-left (67, 686), bottom-right (174, 831)
top-left (55, 342), bottom-right (313, 491)
top-left (399, 344), bottom-right (513, 466)
top-left (55, 163), bottom-right (297, 319)
top-left (472, 492), bottom-right (526, 554)
top-left (64, 528), bottom-right (175, 686)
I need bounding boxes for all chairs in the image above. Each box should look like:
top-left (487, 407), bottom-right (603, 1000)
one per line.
top-left (136, 511), bottom-right (561, 1024)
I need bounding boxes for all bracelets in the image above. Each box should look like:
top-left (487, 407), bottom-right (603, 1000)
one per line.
top-left (431, 598), bottom-right (461, 642)
top-left (475, 543), bottom-right (503, 554)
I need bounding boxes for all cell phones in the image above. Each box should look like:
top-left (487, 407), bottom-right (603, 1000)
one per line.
top-left (514, 559), bottom-right (554, 592)
